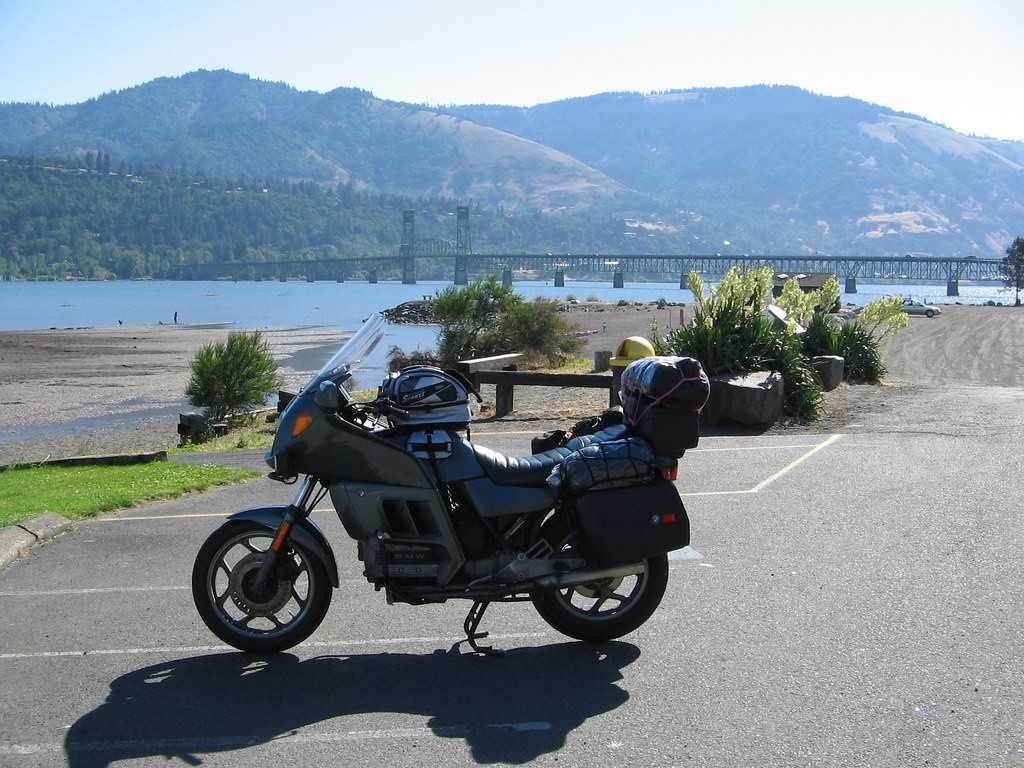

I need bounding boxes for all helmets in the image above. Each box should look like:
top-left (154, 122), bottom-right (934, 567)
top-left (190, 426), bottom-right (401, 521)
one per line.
top-left (379, 364), bottom-right (472, 431)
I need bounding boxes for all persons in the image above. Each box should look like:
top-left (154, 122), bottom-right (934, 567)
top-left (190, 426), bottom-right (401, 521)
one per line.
top-left (174, 312), bottom-right (177, 324)
top-left (602, 321), bottom-right (608, 332)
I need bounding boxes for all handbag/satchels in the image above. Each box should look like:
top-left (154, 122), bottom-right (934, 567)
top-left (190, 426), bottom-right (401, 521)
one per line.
top-left (572, 478), bottom-right (690, 571)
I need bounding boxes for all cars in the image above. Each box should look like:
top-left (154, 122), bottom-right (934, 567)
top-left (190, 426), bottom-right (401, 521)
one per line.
top-left (898, 298), bottom-right (942, 318)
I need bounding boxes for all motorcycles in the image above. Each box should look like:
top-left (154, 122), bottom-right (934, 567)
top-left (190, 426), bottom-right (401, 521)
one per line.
top-left (189, 313), bottom-right (705, 649)
